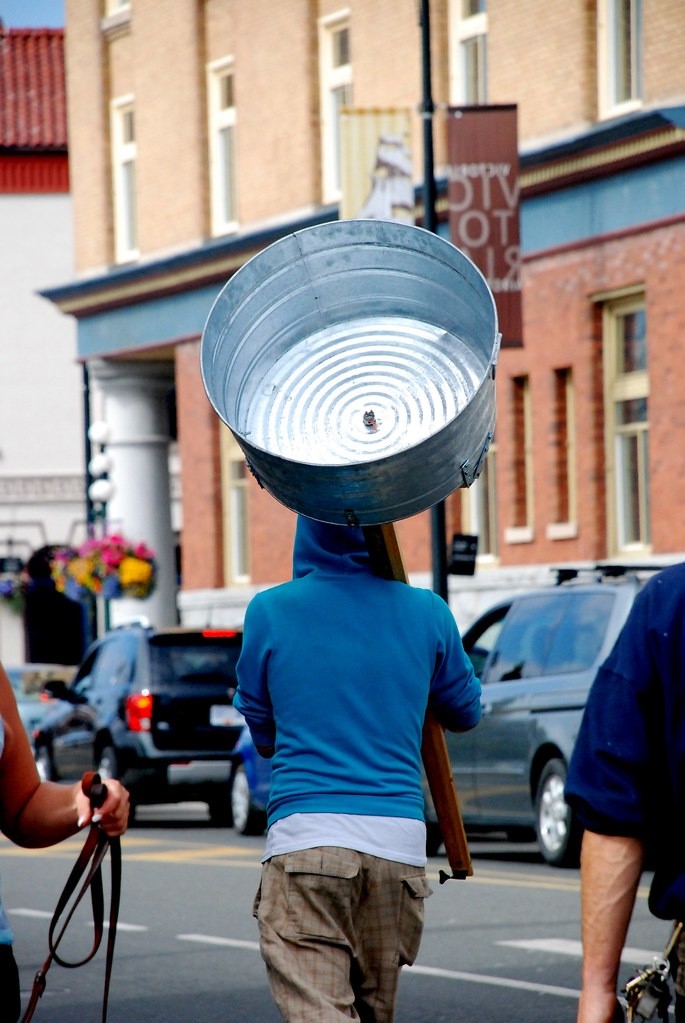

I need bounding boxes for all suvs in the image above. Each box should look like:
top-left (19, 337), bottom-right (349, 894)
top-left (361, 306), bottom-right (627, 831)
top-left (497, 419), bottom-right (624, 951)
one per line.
top-left (420, 562), bottom-right (672, 868)
top-left (32, 618), bottom-right (243, 828)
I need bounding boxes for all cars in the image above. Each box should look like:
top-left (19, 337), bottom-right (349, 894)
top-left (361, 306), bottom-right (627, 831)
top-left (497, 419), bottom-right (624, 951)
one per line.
top-left (230, 725), bottom-right (271, 839)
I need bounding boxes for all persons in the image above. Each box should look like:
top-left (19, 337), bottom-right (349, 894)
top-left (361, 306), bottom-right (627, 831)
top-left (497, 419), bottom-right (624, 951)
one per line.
top-left (0, 662), bottom-right (130, 1023)
top-left (233, 514), bottom-right (482, 1023)
top-left (564, 562), bottom-right (685, 1023)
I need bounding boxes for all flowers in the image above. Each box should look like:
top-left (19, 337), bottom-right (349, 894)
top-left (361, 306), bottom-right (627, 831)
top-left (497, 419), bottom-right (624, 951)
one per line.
top-left (51, 535), bottom-right (152, 604)
top-left (0, 572), bottom-right (34, 596)
